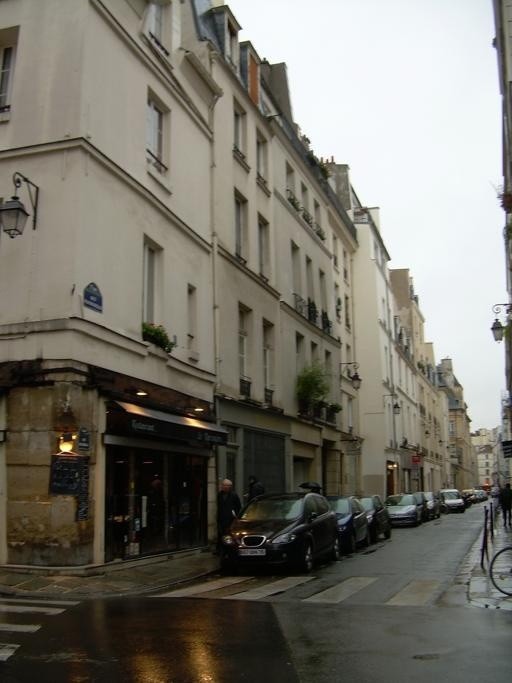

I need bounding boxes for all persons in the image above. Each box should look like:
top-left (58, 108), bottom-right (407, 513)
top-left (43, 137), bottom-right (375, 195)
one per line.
top-left (498, 482), bottom-right (511, 527)
top-left (310, 488), bottom-right (320, 494)
top-left (148, 478), bottom-right (175, 536)
top-left (217, 478), bottom-right (244, 574)
top-left (243, 475), bottom-right (265, 501)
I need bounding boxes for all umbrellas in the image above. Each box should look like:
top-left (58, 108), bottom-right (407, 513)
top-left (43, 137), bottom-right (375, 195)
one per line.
top-left (296, 481), bottom-right (322, 489)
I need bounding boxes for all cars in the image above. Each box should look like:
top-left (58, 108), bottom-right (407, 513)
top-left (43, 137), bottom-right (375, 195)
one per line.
top-left (219, 491), bottom-right (340, 573)
top-left (462, 489), bottom-right (489, 502)
top-left (383, 491), bottom-right (441, 525)
top-left (356, 493), bottom-right (391, 543)
top-left (438, 488), bottom-right (465, 514)
top-left (325, 495), bottom-right (370, 554)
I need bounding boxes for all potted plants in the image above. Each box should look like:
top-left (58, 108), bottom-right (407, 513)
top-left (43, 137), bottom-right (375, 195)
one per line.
top-left (297, 359), bottom-right (343, 424)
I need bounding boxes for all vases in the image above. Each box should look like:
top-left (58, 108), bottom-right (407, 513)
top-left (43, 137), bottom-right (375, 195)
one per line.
top-left (143, 332), bottom-right (165, 348)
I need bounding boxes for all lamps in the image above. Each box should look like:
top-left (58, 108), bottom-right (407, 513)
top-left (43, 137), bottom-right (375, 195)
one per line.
top-left (0, 171), bottom-right (39, 239)
top-left (123, 388), bottom-right (148, 397)
top-left (392, 461), bottom-right (398, 468)
top-left (183, 405), bottom-right (205, 413)
top-left (383, 393), bottom-right (400, 415)
top-left (491, 303), bottom-right (512, 344)
top-left (339, 362), bottom-right (362, 391)
top-left (425, 430), bottom-right (451, 450)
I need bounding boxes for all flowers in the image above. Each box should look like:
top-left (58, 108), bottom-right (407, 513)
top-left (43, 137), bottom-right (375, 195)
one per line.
top-left (142, 321), bottom-right (175, 352)
